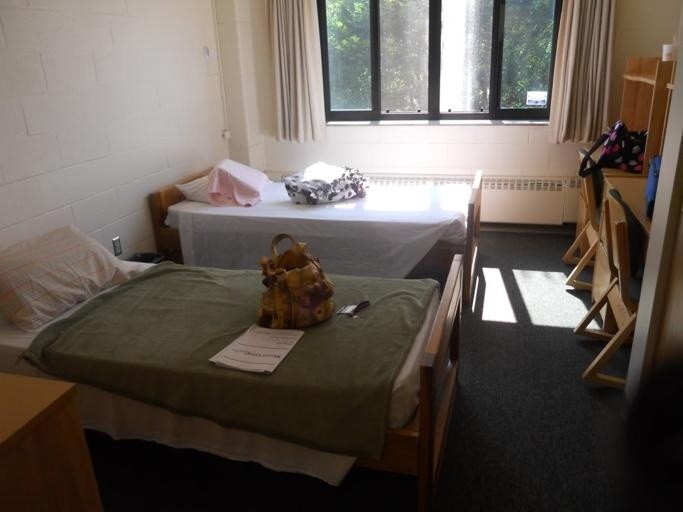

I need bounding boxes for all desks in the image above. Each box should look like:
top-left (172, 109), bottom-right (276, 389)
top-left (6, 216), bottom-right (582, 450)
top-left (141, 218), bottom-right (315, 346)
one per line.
top-left (0, 373), bottom-right (104, 512)
top-left (577, 55), bottom-right (679, 391)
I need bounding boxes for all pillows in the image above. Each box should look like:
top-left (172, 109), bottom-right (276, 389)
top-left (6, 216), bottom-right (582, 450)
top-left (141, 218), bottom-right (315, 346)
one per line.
top-left (175, 174), bottom-right (213, 202)
top-left (1, 225), bottom-right (129, 333)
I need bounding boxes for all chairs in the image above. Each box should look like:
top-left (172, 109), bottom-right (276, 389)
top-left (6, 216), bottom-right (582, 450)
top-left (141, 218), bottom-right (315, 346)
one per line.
top-left (574, 190), bottom-right (640, 391)
top-left (562, 148), bottom-right (601, 288)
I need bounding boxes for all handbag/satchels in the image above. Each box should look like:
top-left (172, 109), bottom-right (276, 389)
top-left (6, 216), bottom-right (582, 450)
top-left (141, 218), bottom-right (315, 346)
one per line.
top-left (578, 122), bottom-right (647, 177)
top-left (258, 234), bottom-right (333, 328)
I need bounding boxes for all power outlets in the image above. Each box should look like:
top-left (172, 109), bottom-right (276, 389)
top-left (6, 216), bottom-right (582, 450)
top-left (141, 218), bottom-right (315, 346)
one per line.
top-left (112, 236), bottom-right (122, 256)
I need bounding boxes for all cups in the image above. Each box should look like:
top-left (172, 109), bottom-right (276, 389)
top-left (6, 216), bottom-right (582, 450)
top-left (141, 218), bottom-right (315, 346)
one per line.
top-left (662, 45), bottom-right (680, 63)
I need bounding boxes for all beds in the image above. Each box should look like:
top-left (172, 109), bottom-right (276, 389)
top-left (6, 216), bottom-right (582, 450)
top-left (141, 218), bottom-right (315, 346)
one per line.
top-left (0, 224), bottom-right (464, 512)
top-left (148, 159), bottom-right (493, 303)
